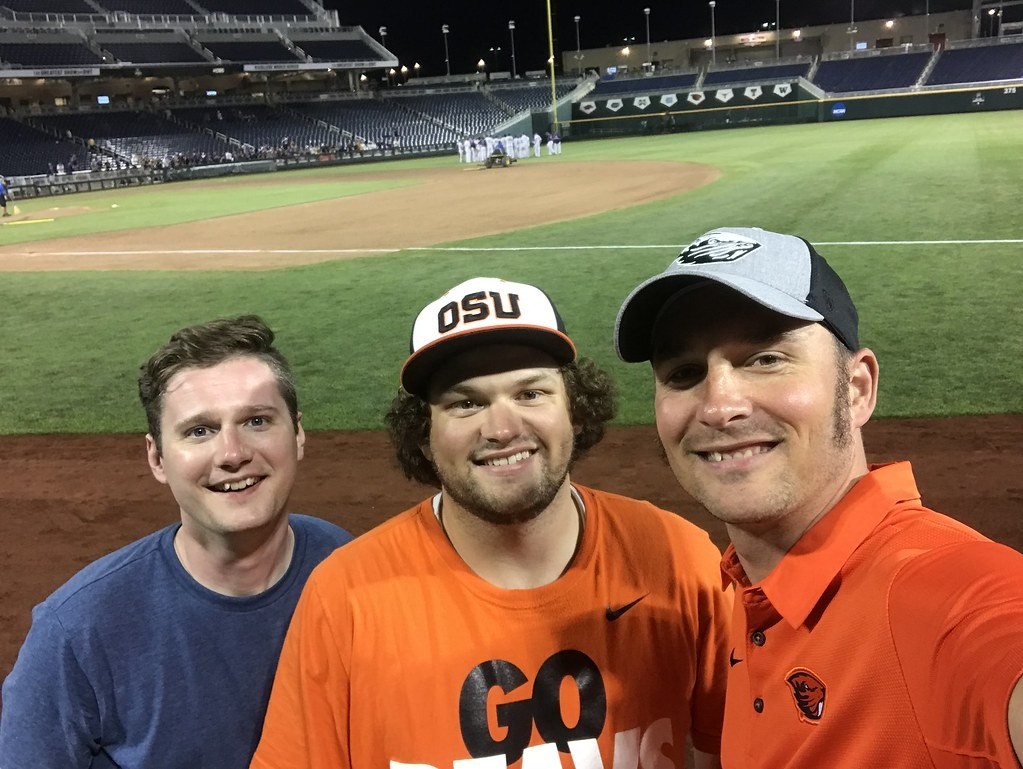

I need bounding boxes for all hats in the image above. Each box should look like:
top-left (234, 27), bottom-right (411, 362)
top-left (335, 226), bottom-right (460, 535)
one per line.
top-left (400, 276), bottom-right (576, 395)
top-left (613, 227), bottom-right (858, 364)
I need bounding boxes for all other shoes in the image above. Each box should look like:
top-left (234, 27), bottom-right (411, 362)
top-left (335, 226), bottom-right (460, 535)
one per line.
top-left (3, 213), bottom-right (10, 216)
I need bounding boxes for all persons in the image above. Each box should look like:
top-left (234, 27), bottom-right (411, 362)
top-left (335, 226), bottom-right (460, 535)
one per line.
top-left (553, 134), bottom-right (562, 155)
top-left (106, 138), bottom-right (111, 149)
top-left (90, 159), bottom-right (163, 172)
top-left (251, 274), bottom-right (735, 769)
top-left (218, 110), bottom-right (222, 120)
top-left (457, 132), bottom-right (530, 163)
top-left (394, 139), bottom-right (400, 152)
top-left (0, 317), bottom-right (356, 769)
top-left (164, 108), bottom-right (171, 120)
top-left (616, 227), bottom-right (1023, 769)
top-left (169, 137), bottom-right (377, 171)
top-left (547, 132), bottom-right (556, 155)
top-left (65, 129), bottom-right (72, 140)
top-left (533, 133), bottom-right (542, 157)
top-left (495, 141), bottom-right (506, 154)
top-left (659, 55), bottom-right (753, 75)
top-left (726, 111), bottom-right (736, 128)
top-left (0, 175), bottom-right (11, 217)
top-left (47, 152), bottom-right (77, 175)
top-left (87, 136), bottom-right (96, 150)
top-left (641, 119), bottom-right (654, 136)
